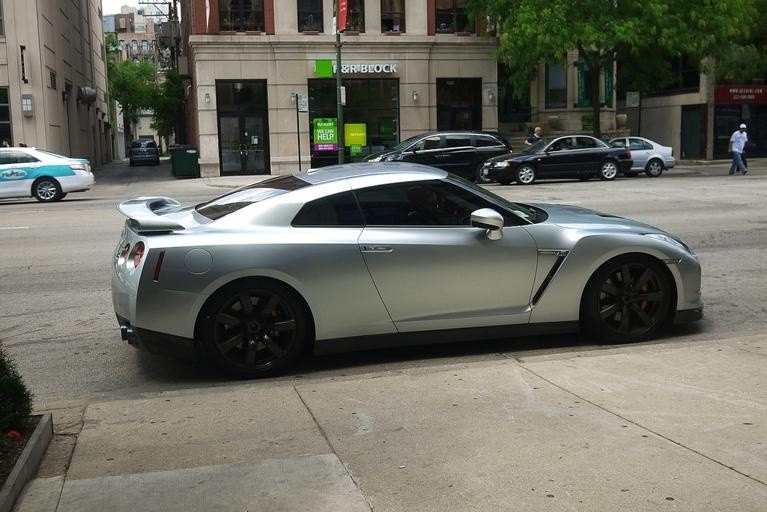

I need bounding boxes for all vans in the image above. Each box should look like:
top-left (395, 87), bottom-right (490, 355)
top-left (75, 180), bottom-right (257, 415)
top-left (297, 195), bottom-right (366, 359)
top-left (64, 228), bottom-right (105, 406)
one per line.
top-left (128, 139), bottom-right (161, 165)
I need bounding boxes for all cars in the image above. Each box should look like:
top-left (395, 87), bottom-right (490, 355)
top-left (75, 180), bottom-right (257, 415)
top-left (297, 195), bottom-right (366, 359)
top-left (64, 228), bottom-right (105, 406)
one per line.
top-left (479, 133), bottom-right (632, 184)
top-left (606, 135), bottom-right (676, 178)
top-left (0, 145), bottom-right (97, 204)
top-left (362, 130), bottom-right (513, 185)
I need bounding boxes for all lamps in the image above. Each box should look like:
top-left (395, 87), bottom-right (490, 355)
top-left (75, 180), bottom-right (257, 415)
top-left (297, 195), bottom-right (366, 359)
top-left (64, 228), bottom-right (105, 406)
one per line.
top-left (205, 93), bottom-right (209, 102)
top-left (291, 92), bottom-right (296, 103)
top-left (412, 91), bottom-right (417, 100)
top-left (489, 90), bottom-right (493, 99)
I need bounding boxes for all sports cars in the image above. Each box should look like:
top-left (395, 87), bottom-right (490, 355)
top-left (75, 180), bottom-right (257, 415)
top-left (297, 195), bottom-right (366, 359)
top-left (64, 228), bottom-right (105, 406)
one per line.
top-left (111, 159), bottom-right (707, 380)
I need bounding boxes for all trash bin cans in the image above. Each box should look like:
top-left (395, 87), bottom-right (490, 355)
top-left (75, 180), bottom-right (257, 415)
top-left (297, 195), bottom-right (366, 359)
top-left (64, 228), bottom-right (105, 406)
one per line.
top-left (168, 144), bottom-right (200, 179)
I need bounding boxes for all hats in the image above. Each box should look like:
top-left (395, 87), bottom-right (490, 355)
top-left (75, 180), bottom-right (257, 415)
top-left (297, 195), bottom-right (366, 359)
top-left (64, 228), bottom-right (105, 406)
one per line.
top-left (739, 124), bottom-right (747, 129)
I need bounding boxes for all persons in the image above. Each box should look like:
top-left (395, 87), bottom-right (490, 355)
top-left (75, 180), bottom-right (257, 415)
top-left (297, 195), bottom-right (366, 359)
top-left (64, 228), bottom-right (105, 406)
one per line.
top-left (728, 123), bottom-right (748, 174)
top-left (524, 127), bottom-right (543, 146)
top-left (2, 139), bottom-right (10, 147)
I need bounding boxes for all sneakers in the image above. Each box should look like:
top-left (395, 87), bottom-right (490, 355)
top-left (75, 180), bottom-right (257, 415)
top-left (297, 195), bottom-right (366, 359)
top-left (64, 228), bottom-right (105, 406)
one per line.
top-left (728, 170), bottom-right (749, 176)
